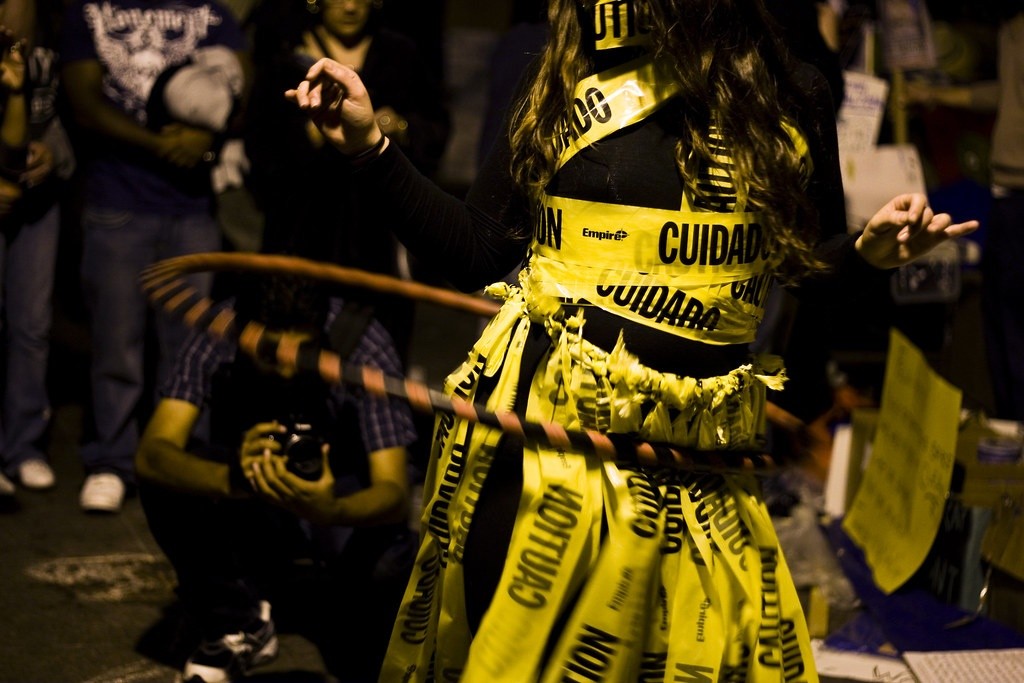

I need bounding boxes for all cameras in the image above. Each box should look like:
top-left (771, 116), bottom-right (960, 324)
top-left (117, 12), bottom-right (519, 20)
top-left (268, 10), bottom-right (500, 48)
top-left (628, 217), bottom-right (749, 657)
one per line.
top-left (268, 424), bottom-right (326, 482)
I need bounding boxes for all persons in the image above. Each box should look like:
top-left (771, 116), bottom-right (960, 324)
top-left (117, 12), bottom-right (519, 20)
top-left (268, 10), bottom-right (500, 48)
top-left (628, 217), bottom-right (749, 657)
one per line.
top-left (0, 0), bottom-right (1024, 682)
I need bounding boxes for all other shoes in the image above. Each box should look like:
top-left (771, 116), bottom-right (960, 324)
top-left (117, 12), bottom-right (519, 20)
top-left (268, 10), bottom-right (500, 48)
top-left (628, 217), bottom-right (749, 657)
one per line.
top-left (80, 472), bottom-right (125, 510)
top-left (20, 461), bottom-right (56, 488)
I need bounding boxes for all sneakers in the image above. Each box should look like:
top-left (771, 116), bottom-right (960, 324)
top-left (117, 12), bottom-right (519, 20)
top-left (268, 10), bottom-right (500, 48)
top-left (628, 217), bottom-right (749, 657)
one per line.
top-left (182, 609), bottom-right (279, 683)
